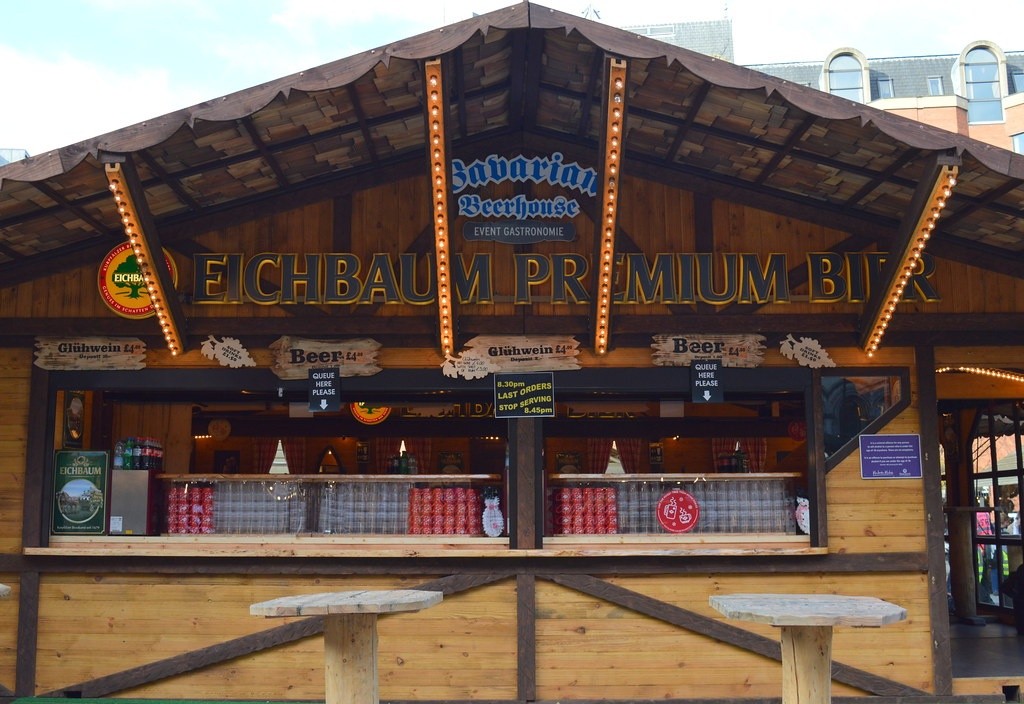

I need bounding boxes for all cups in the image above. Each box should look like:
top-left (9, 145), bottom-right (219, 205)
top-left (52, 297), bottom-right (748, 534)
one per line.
top-left (554, 480), bottom-right (791, 533)
top-left (166, 479), bottom-right (480, 533)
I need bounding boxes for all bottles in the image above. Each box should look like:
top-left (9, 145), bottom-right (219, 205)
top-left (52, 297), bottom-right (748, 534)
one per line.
top-left (113, 436), bottom-right (162, 472)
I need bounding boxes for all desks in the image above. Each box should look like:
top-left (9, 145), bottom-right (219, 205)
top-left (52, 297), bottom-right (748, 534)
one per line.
top-left (709, 594), bottom-right (906, 704)
top-left (249, 589), bottom-right (444, 704)
